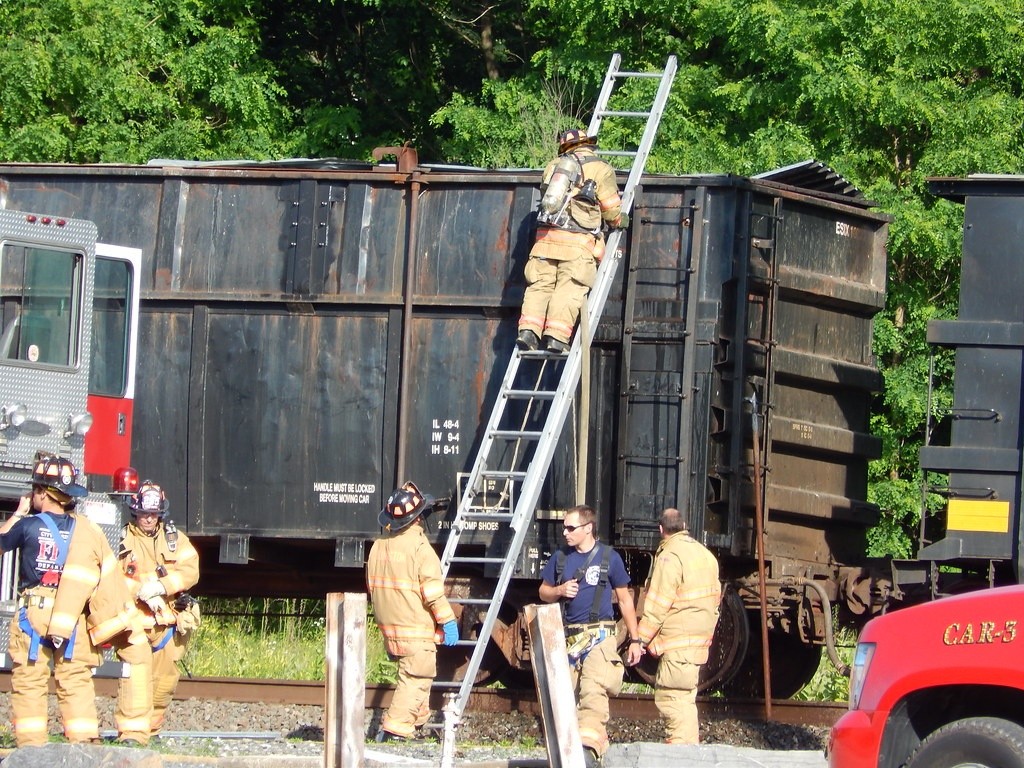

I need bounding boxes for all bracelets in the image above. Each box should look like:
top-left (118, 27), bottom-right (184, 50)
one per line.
top-left (631, 638), bottom-right (639, 643)
top-left (14, 511), bottom-right (24, 518)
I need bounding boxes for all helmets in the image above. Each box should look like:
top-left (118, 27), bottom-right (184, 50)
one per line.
top-left (127, 479), bottom-right (169, 512)
top-left (25, 454), bottom-right (88, 497)
top-left (558, 127), bottom-right (597, 154)
top-left (377, 481), bottom-right (427, 530)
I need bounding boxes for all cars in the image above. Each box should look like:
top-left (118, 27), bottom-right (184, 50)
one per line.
top-left (823, 584), bottom-right (1024, 768)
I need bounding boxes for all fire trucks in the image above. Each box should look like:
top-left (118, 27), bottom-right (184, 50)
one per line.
top-left (0, 208), bottom-right (144, 566)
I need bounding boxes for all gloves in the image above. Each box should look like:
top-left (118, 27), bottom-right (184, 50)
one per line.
top-left (136, 579), bottom-right (166, 601)
top-left (617, 211), bottom-right (629, 230)
top-left (143, 594), bottom-right (166, 613)
top-left (442, 620), bottom-right (459, 646)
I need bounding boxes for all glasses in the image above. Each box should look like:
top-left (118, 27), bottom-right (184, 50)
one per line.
top-left (563, 522), bottom-right (591, 531)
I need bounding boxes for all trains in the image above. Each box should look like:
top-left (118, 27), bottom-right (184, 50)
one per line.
top-left (0, 142), bottom-right (1024, 694)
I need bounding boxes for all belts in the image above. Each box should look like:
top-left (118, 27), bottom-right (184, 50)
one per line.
top-left (17, 594), bottom-right (55, 609)
top-left (564, 622), bottom-right (615, 637)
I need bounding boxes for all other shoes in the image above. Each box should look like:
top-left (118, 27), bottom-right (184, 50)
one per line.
top-left (151, 735), bottom-right (160, 744)
top-left (122, 738), bottom-right (138, 747)
top-left (388, 734), bottom-right (423, 745)
top-left (516, 331), bottom-right (539, 351)
top-left (546, 336), bottom-right (570, 352)
top-left (582, 746), bottom-right (601, 768)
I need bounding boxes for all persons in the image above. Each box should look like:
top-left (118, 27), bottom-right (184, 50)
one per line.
top-left (366, 481), bottom-right (460, 743)
top-left (539, 504), bottom-right (640, 768)
top-left (107, 480), bottom-right (199, 749)
top-left (515, 130), bottom-right (630, 352)
top-left (638, 508), bottom-right (722, 744)
top-left (0, 449), bottom-right (104, 749)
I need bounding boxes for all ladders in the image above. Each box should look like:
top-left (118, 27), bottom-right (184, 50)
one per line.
top-left (352, 53), bottom-right (677, 768)
